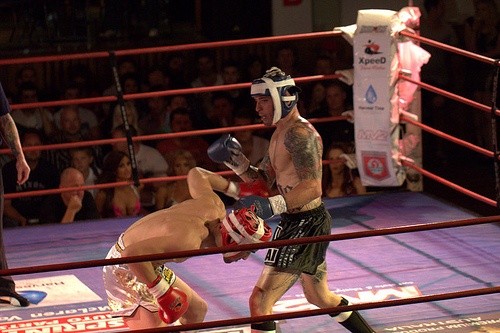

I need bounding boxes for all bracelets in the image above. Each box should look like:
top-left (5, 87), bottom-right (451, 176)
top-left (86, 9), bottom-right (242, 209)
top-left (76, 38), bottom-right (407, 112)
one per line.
top-left (147, 276), bottom-right (162, 288)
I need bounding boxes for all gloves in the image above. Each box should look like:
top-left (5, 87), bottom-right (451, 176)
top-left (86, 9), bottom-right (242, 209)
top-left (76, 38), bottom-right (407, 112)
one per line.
top-left (234, 195), bottom-right (287, 220)
top-left (207, 134), bottom-right (252, 177)
top-left (147, 273), bottom-right (188, 324)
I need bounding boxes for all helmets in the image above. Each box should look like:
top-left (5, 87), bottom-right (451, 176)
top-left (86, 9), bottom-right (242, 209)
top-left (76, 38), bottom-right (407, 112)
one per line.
top-left (221, 209), bottom-right (273, 258)
top-left (250, 67), bottom-right (299, 127)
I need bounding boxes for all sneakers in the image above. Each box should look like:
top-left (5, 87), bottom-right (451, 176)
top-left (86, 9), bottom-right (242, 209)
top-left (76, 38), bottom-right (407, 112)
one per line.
top-left (0, 292), bottom-right (28, 307)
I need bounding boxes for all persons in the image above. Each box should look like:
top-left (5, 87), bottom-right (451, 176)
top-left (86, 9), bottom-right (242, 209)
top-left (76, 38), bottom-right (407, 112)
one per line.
top-left (104, 168), bottom-right (271, 333)
top-left (0, 81), bottom-right (31, 306)
top-left (0, 0), bottom-right (500, 225)
top-left (209, 69), bottom-right (378, 333)
